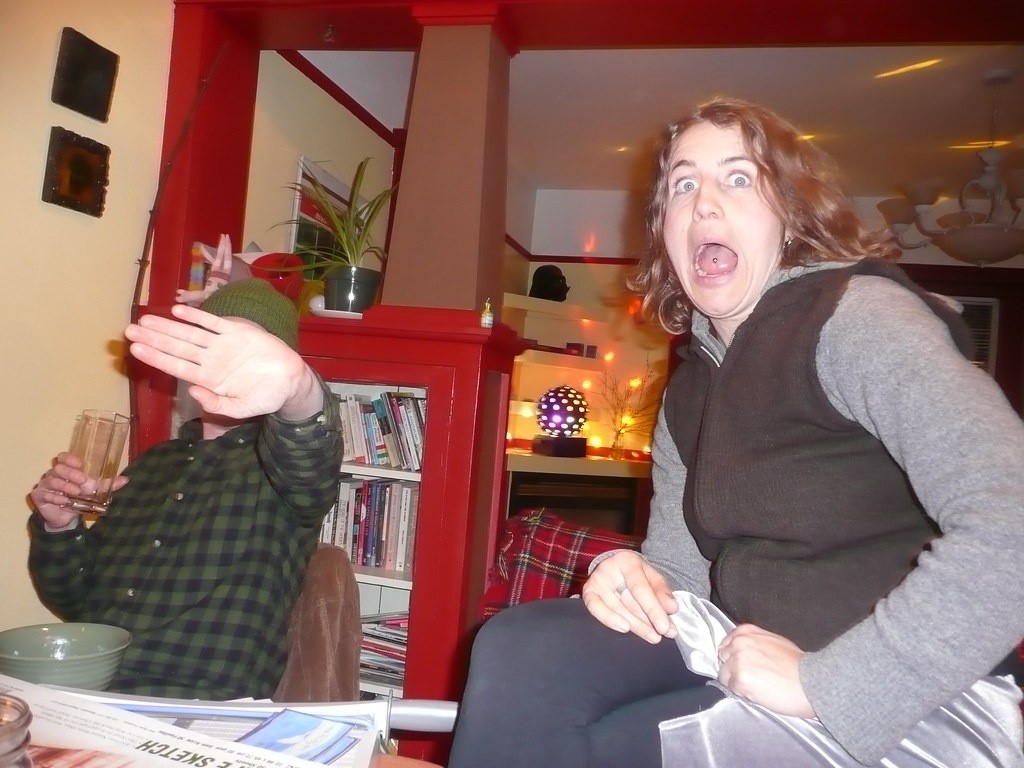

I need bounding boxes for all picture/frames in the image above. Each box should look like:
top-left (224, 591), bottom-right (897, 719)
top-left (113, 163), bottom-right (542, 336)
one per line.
top-left (41, 124), bottom-right (113, 218)
top-left (50, 26), bottom-right (121, 122)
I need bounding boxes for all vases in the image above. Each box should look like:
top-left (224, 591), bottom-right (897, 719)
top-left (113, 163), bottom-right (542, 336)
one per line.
top-left (612, 432), bottom-right (623, 458)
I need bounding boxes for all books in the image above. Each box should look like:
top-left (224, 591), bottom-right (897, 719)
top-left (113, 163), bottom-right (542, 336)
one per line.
top-left (318, 473), bottom-right (420, 572)
top-left (361, 611), bottom-right (410, 686)
top-left (317, 391), bottom-right (428, 471)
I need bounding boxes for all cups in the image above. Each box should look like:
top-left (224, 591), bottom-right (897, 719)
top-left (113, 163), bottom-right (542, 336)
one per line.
top-left (0, 693), bottom-right (34, 768)
top-left (59, 409), bottom-right (129, 520)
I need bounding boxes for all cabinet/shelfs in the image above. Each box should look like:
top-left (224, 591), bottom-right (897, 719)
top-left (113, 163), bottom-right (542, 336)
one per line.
top-left (503, 292), bottom-right (652, 516)
top-left (129, 303), bottom-right (517, 759)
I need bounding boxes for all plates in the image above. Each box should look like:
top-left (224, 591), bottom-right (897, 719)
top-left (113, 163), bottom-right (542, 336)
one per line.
top-left (310, 309), bottom-right (362, 318)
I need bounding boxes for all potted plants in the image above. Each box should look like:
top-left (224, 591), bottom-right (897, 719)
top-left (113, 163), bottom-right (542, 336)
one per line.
top-left (265, 154), bottom-right (401, 312)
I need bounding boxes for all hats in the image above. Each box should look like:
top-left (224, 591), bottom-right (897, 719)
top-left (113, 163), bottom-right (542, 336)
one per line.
top-left (199, 277), bottom-right (299, 352)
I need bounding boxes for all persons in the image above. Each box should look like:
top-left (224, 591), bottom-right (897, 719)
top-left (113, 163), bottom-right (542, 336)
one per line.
top-left (448, 98), bottom-right (1024, 768)
top-left (27, 278), bottom-right (344, 700)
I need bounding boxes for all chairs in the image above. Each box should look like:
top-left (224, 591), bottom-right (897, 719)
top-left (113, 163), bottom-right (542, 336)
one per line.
top-left (272, 542), bottom-right (360, 703)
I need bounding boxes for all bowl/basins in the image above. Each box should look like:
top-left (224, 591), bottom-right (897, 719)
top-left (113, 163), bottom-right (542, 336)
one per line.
top-left (0, 623), bottom-right (132, 691)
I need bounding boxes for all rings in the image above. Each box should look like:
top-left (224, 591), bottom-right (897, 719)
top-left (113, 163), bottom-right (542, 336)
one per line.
top-left (717, 648), bottom-right (726, 664)
top-left (614, 581), bottom-right (627, 599)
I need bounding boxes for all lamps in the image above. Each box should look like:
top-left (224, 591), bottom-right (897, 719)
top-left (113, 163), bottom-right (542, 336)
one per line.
top-left (874, 68), bottom-right (1024, 269)
top-left (531, 386), bottom-right (589, 458)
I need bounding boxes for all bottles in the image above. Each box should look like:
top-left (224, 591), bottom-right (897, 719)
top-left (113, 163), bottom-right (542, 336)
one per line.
top-left (481, 303), bottom-right (493, 328)
